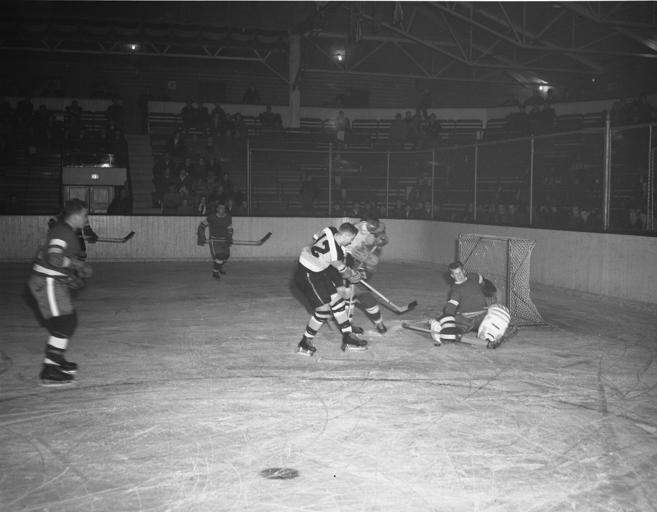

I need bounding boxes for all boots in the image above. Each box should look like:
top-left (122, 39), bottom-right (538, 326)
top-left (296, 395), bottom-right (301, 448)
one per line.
top-left (343, 326), bottom-right (367, 347)
top-left (211, 267), bottom-right (226, 279)
top-left (439, 329), bottom-right (462, 345)
top-left (374, 320), bottom-right (387, 333)
top-left (298, 334), bottom-right (317, 352)
top-left (39, 356), bottom-right (79, 380)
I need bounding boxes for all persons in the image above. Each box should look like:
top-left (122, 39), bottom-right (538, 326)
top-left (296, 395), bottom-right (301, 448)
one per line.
top-left (439, 262), bottom-right (511, 348)
top-left (198, 204), bottom-right (233, 280)
top-left (153, 99), bottom-right (282, 208)
top-left (0, 94), bottom-right (125, 165)
top-left (300, 94), bottom-right (657, 232)
top-left (27, 198), bottom-right (99, 382)
top-left (295, 216), bottom-right (388, 351)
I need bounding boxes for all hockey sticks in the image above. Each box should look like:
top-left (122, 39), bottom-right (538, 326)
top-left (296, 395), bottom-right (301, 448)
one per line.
top-left (360, 279), bottom-right (417, 312)
top-left (77, 231), bottom-right (134, 242)
top-left (204, 232), bottom-right (272, 245)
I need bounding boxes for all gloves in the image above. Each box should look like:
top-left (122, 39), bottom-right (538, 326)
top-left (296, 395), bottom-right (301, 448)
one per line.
top-left (69, 258), bottom-right (91, 278)
top-left (197, 234), bottom-right (206, 246)
top-left (338, 265), bottom-right (367, 283)
top-left (226, 236), bottom-right (232, 244)
top-left (85, 232), bottom-right (99, 244)
